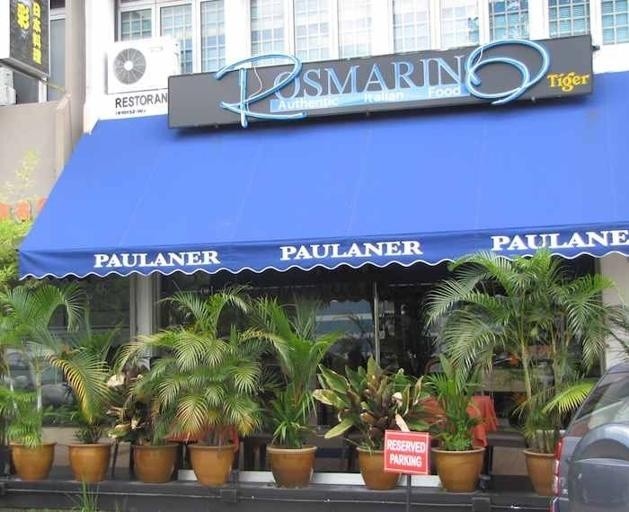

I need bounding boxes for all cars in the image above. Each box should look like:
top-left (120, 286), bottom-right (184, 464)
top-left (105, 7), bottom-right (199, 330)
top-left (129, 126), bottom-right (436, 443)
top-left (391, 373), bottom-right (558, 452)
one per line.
top-left (5, 346), bottom-right (76, 408)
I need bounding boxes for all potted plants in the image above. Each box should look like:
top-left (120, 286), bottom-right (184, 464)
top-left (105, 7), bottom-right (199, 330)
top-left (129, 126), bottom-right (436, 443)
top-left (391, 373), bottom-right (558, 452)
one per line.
top-left (1, 279), bottom-right (89, 482)
top-left (66, 310), bottom-right (124, 483)
top-left (107, 374), bottom-right (179, 483)
top-left (313, 353), bottom-right (425, 490)
top-left (422, 248), bottom-right (625, 497)
top-left (427, 353), bottom-right (493, 492)
top-left (116, 281), bottom-right (293, 487)
top-left (235, 292), bottom-right (352, 487)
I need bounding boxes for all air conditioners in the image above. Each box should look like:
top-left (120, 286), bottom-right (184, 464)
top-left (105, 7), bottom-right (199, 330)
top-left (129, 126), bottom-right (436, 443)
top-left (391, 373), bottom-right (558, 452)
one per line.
top-left (105, 35), bottom-right (181, 94)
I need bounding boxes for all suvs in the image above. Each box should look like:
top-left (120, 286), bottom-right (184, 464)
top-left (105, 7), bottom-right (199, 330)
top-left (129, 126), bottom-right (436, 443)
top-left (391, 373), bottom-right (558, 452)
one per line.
top-left (544, 362), bottom-right (628, 512)
